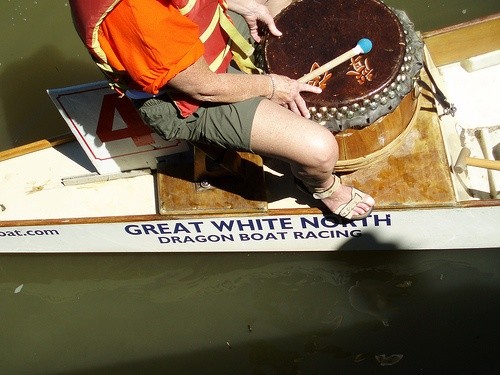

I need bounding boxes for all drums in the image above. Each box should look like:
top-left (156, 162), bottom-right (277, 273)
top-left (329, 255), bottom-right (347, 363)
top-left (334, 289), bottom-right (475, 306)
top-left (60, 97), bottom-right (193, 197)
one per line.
top-left (252, 0), bottom-right (419, 173)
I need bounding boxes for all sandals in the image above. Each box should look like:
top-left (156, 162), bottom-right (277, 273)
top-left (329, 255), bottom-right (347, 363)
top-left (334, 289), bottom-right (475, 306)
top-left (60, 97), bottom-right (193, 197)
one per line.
top-left (294, 174), bottom-right (375, 221)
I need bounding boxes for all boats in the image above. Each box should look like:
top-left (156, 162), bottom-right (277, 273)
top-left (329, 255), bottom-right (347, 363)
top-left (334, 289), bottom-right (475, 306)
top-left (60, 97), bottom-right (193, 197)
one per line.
top-left (0, 12), bottom-right (500, 255)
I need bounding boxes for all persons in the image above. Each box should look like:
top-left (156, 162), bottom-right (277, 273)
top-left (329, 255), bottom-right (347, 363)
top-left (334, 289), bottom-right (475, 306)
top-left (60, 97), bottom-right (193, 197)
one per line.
top-left (72, 0), bottom-right (375, 220)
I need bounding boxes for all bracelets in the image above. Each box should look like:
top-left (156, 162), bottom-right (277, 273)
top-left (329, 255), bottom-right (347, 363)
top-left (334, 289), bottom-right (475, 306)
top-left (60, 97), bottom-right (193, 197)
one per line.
top-left (265, 75), bottom-right (276, 101)
